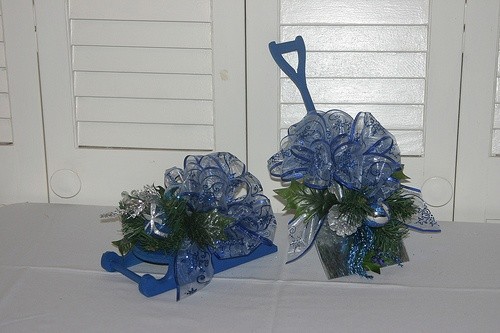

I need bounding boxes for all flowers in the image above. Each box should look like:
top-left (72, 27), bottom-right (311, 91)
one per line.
top-left (101, 152), bottom-right (278, 301)
top-left (268, 107), bottom-right (442, 279)
